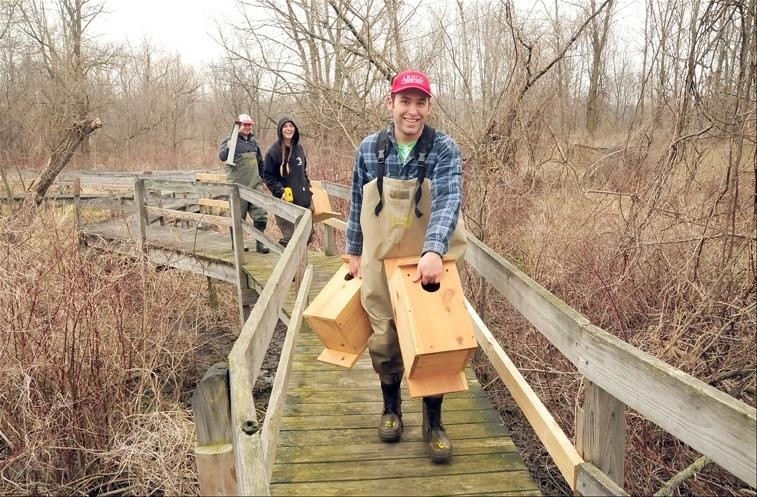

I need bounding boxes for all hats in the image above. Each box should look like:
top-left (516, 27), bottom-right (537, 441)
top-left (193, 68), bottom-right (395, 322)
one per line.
top-left (237, 113), bottom-right (254, 125)
top-left (393, 70), bottom-right (432, 98)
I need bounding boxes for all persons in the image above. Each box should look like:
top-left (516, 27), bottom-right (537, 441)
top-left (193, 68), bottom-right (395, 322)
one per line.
top-left (217, 113), bottom-right (271, 254)
top-left (344, 69), bottom-right (469, 465)
top-left (263, 117), bottom-right (315, 282)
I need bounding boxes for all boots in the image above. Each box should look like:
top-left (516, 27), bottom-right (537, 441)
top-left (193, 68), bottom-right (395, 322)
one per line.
top-left (254, 221), bottom-right (270, 253)
top-left (380, 380), bottom-right (403, 440)
top-left (423, 395), bottom-right (451, 465)
top-left (230, 227), bottom-right (248, 252)
